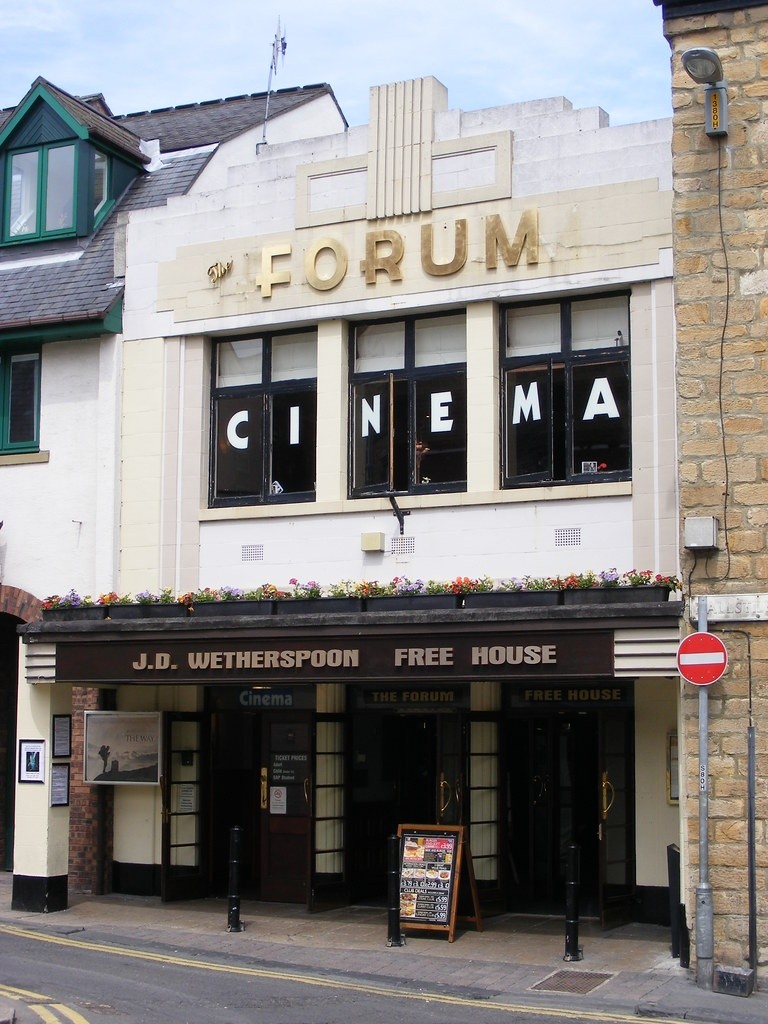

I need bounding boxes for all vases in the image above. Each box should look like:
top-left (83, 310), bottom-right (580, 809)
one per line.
top-left (42, 584), bottom-right (672, 621)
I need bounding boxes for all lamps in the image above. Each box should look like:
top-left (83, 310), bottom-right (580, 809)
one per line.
top-left (681, 46), bottom-right (728, 137)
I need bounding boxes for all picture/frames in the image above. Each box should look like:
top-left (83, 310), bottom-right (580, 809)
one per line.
top-left (18, 739), bottom-right (46, 783)
top-left (53, 714), bottom-right (71, 758)
top-left (83, 710), bottom-right (163, 787)
top-left (49, 763), bottom-right (71, 807)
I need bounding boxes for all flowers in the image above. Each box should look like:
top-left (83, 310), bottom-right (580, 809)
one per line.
top-left (38, 568), bottom-right (686, 610)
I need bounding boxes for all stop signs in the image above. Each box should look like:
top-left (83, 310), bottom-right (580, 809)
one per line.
top-left (676, 632), bottom-right (727, 685)
